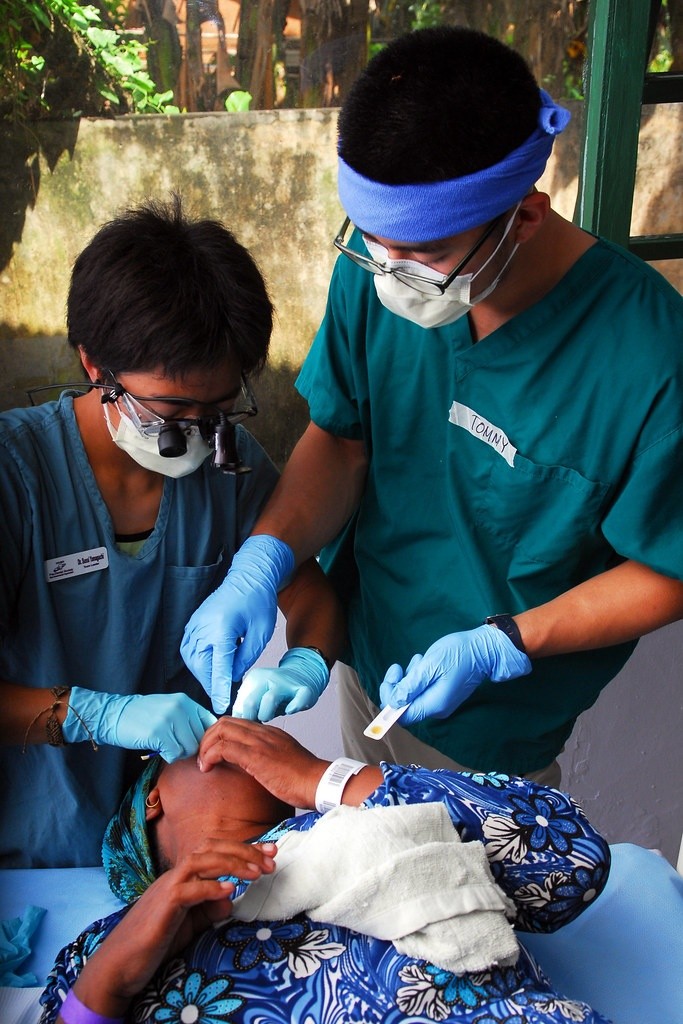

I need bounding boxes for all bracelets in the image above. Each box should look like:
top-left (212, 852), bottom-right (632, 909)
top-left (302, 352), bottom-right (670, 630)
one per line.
top-left (21, 685), bottom-right (99, 756)
top-left (58, 985), bottom-right (126, 1024)
top-left (481, 610), bottom-right (529, 660)
top-left (313, 755), bottom-right (371, 821)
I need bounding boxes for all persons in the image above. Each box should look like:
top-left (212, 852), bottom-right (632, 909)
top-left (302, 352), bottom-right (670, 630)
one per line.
top-left (31, 710), bottom-right (617, 1023)
top-left (177, 22), bottom-right (683, 824)
top-left (0, 203), bottom-right (342, 873)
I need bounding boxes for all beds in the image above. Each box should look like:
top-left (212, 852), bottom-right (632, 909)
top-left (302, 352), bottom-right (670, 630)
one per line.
top-left (0, 843), bottom-right (683, 1024)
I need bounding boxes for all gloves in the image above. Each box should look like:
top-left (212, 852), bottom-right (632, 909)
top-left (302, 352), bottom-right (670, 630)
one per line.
top-left (232, 648), bottom-right (329, 721)
top-left (178, 534), bottom-right (295, 715)
top-left (381, 622), bottom-right (531, 726)
top-left (62, 685), bottom-right (218, 765)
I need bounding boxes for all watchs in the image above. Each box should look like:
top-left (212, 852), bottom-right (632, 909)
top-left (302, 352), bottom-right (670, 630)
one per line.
top-left (304, 644), bottom-right (328, 664)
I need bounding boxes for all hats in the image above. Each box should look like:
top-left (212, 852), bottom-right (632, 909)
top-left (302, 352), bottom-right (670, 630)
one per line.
top-left (102, 752), bottom-right (159, 901)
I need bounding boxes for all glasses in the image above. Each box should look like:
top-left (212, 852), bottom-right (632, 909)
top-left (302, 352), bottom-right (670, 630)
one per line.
top-left (109, 368), bottom-right (259, 440)
top-left (333, 209), bottom-right (508, 297)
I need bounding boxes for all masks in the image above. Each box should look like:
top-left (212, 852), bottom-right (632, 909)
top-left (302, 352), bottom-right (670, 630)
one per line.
top-left (100, 368), bottom-right (244, 478)
top-left (362, 189), bottom-right (525, 328)
